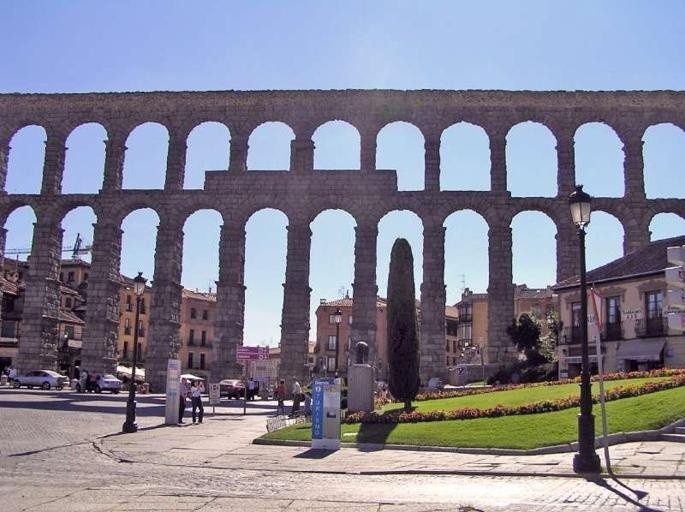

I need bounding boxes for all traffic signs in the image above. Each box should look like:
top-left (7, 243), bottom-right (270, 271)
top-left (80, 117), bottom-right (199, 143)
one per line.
top-left (562, 355), bottom-right (600, 364)
top-left (235, 353), bottom-right (269, 360)
top-left (234, 345), bottom-right (269, 354)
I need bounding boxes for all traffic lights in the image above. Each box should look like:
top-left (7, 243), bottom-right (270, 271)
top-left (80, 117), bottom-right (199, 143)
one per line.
top-left (661, 244), bottom-right (685, 333)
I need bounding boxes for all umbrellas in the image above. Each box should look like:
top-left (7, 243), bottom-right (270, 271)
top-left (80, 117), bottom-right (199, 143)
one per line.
top-left (180, 374), bottom-right (205, 384)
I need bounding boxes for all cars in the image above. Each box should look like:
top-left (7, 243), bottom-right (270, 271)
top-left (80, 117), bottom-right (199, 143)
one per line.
top-left (72, 374), bottom-right (124, 394)
top-left (217, 378), bottom-right (276, 400)
top-left (9, 368), bottom-right (70, 392)
top-left (297, 377), bottom-right (312, 400)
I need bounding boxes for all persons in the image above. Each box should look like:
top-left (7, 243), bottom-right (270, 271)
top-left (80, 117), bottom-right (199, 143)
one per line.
top-left (4, 366), bottom-right (16, 382)
top-left (274, 379), bottom-right (285, 415)
top-left (248, 378), bottom-right (255, 400)
top-left (288, 377), bottom-right (301, 417)
top-left (178, 378), bottom-right (188, 424)
top-left (191, 381), bottom-right (205, 424)
top-left (79, 369), bottom-right (97, 392)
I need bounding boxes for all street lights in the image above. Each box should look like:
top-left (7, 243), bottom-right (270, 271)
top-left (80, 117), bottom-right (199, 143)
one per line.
top-left (120, 266), bottom-right (149, 433)
top-left (563, 180), bottom-right (600, 472)
top-left (333, 308), bottom-right (342, 378)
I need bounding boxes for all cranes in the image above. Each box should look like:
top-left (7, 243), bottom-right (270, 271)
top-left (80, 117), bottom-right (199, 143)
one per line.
top-left (4, 233), bottom-right (94, 260)
